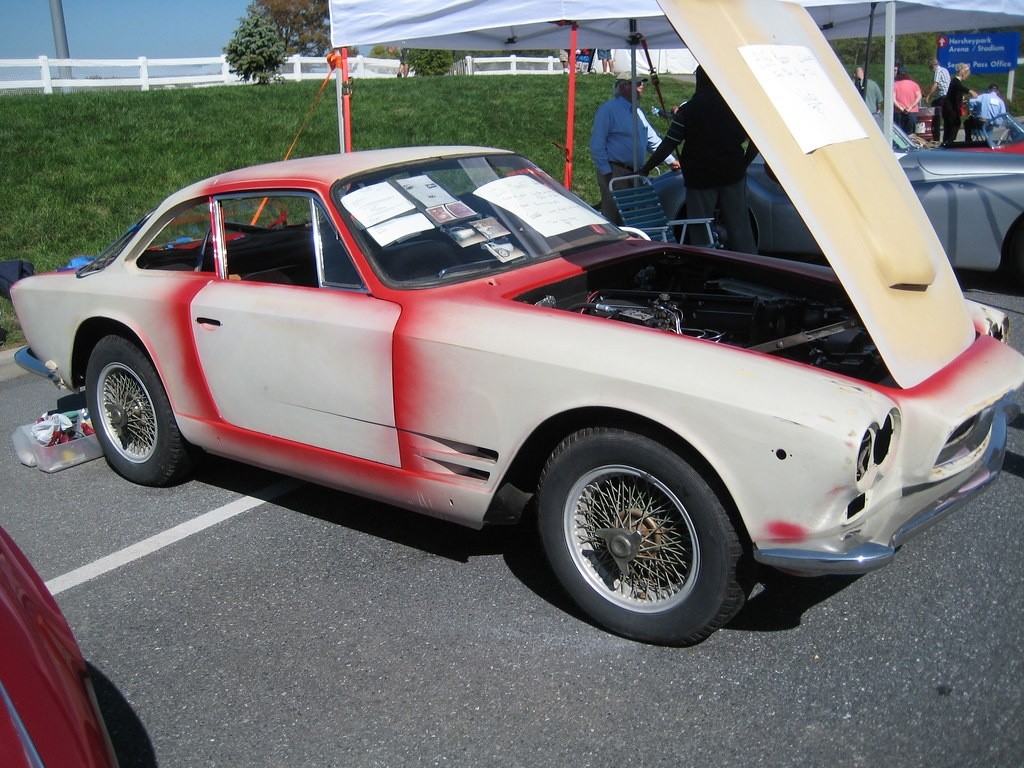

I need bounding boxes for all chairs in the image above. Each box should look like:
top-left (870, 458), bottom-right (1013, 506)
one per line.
top-left (219, 232), bottom-right (459, 284)
top-left (609, 174), bottom-right (717, 249)
top-left (971, 127), bottom-right (994, 140)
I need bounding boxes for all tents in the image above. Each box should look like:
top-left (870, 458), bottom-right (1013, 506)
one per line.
top-left (328, 0), bottom-right (1024, 194)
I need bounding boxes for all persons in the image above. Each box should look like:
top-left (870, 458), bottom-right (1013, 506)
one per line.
top-left (597, 49), bottom-right (615, 74)
top-left (924, 58), bottom-right (951, 144)
top-left (941, 63), bottom-right (978, 146)
top-left (895, 63), bottom-right (901, 75)
top-left (590, 71), bottom-right (680, 225)
top-left (635, 65), bottom-right (759, 255)
top-left (963, 84), bottom-right (1006, 146)
top-left (559, 49), bottom-right (569, 74)
top-left (894, 67), bottom-right (922, 135)
top-left (852, 67), bottom-right (883, 114)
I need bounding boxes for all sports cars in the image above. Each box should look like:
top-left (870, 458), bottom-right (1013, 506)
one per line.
top-left (640, 113), bottom-right (1024, 294)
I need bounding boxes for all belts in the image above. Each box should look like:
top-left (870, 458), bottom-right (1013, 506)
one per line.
top-left (610, 161), bottom-right (636, 173)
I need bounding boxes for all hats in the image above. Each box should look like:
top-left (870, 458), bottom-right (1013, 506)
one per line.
top-left (617, 72), bottom-right (650, 84)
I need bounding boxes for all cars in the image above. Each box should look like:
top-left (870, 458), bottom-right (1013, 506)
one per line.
top-left (2, 538), bottom-right (160, 768)
top-left (8, 0), bottom-right (1024, 650)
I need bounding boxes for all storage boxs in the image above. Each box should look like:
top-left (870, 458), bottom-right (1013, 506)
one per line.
top-left (11, 422), bottom-right (105, 472)
